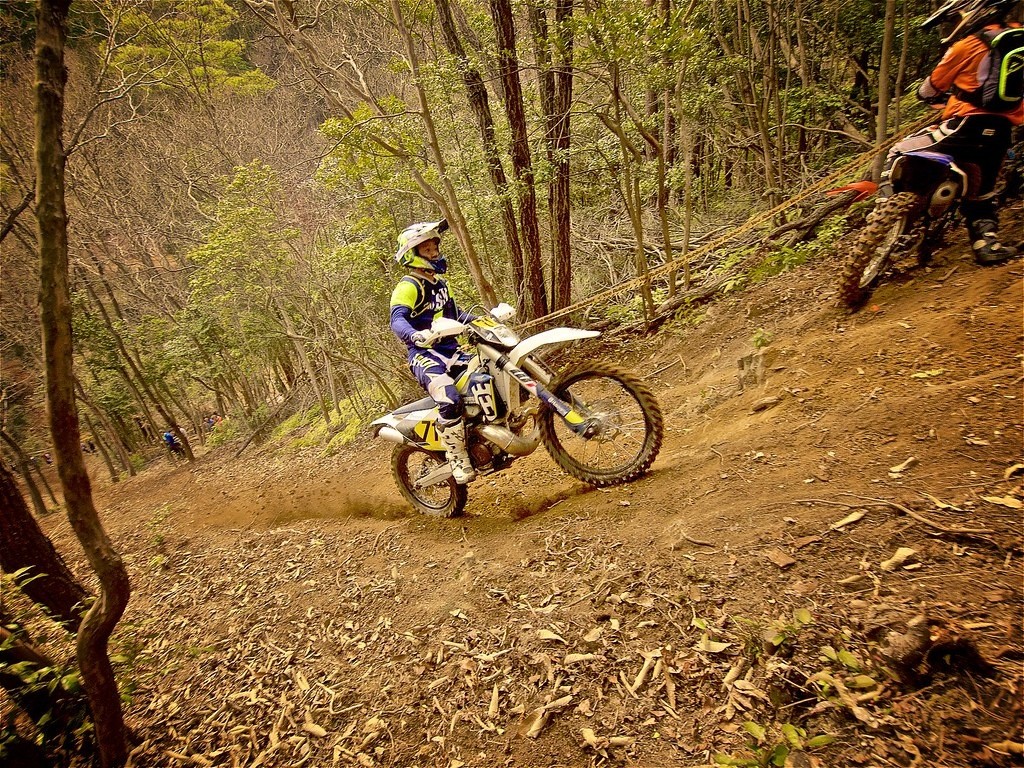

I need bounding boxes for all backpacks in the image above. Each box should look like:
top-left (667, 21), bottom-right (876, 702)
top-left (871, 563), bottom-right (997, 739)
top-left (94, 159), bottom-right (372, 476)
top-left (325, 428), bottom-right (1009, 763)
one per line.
top-left (950, 22), bottom-right (1024, 113)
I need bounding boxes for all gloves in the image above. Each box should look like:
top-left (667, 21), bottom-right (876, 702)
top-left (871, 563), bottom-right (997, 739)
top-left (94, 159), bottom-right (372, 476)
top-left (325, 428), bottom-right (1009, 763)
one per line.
top-left (489, 307), bottom-right (501, 320)
top-left (410, 328), bottom-right (437, 348)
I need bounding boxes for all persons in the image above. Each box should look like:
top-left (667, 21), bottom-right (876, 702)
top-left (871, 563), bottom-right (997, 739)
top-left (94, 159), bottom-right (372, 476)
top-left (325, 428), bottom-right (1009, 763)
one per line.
top-left (159, 410), bottom-right (229, 460)
top-left (886, 0), bottom-right (1024, 265)
top-left (8, 438), bottom-right (99, 475)
top-left (390, 217), bottom-right (508, 486)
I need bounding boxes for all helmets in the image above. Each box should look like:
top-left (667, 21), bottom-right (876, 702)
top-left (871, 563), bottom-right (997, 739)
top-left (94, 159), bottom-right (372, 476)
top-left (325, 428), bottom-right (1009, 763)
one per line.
top-left (919, 0), bottom-right (1022, 45)
top-left (395, 217), bottom-right (449, 274)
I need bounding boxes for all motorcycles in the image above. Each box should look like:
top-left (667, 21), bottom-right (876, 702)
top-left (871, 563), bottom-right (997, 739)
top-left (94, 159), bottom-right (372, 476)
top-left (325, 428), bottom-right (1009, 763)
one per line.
top-left (370, 303), bottom-right (663, 518)
top-left (834, 93), bottom-right (967, 312)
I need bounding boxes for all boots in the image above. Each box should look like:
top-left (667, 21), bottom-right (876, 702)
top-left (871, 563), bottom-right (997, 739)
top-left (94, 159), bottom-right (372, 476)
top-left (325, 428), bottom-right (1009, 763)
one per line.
top-left (434, 417), bottom-right (476, 485)
top-left (969, 219), bottom-right (1019, 264)
top-left (865, 180), bottom-right (895, 225)
top-left (503, 452), bottom-right (517, 462)
